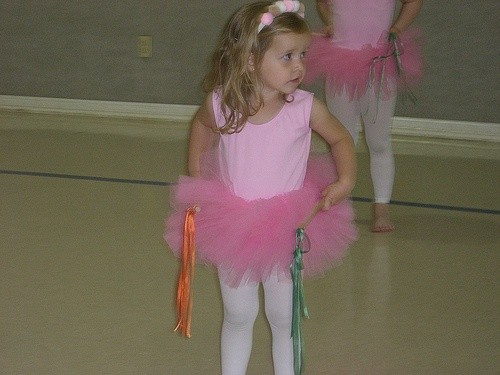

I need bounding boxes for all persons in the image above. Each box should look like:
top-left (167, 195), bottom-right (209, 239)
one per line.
top-left (303, 0), bottom-right (423, 233)
top-left (162, 1), bottom-right (360, 375)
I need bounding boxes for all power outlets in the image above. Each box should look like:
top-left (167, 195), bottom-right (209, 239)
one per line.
top-left (135, 34), bottom-right (152, 58)
